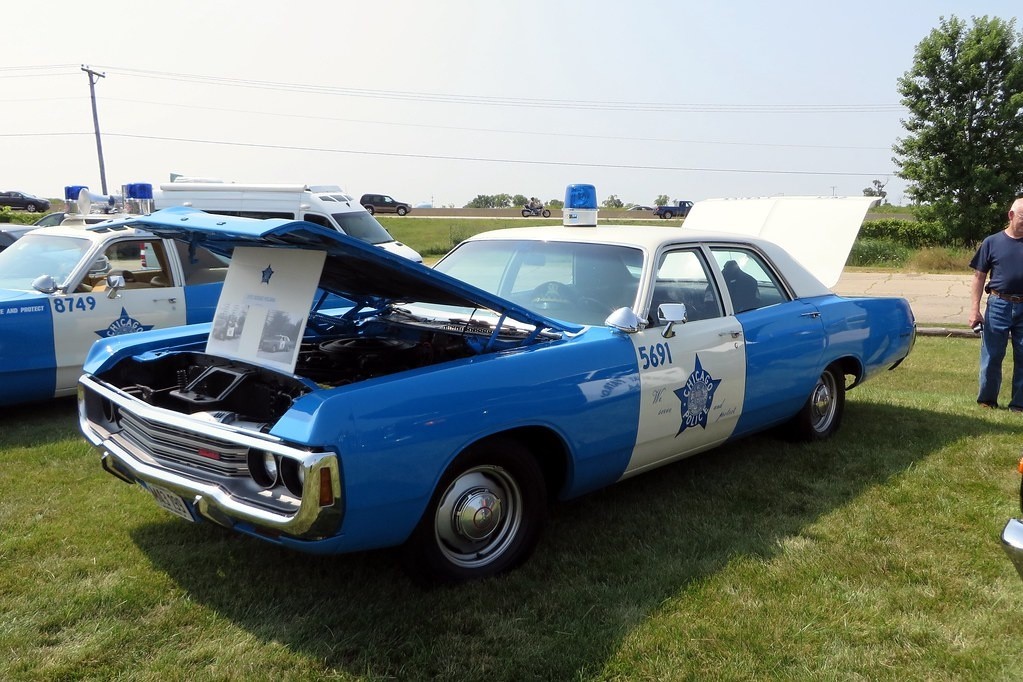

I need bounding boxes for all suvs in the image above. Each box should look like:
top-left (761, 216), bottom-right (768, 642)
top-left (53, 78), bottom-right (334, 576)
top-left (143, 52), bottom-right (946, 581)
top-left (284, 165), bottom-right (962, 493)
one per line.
top-left (360, 194), bottom-right (412, 216)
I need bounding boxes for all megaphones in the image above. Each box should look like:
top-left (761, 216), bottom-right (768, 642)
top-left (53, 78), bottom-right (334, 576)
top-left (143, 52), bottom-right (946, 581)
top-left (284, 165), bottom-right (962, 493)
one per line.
top-left (77, 187), bottom-right (111, 215)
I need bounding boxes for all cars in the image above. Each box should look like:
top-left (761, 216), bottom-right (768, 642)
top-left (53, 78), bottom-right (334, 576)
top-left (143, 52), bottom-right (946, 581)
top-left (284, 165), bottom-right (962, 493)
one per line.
top-left (76, 196), bottom-right (917, 583)
top-left (33, 212), bottom-right (65, 226)
top-left (0, 191), bottom-right (51, 213)
top-left (0, 225), bottom-right (39, 253)
top-left (0, 220), bottom-right (386, 417)
top-left (627, 206), bottom-right (653, 211)
top-left (262, 335), bottom-right (290, 352)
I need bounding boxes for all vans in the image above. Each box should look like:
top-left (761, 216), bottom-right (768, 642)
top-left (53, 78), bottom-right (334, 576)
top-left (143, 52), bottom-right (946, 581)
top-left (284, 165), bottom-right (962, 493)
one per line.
top-left (153, 173), bottom-right (424, 268)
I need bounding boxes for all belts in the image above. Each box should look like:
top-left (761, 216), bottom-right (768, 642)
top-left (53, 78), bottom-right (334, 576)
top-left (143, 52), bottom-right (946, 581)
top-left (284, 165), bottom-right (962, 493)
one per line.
top-left (989, 289), bottom-right (1023, 303)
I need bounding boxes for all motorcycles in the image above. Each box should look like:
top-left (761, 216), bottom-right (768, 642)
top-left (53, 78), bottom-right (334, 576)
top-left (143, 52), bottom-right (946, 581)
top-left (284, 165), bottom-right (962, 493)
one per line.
top-left (522, 204), bottom-right (551, 217)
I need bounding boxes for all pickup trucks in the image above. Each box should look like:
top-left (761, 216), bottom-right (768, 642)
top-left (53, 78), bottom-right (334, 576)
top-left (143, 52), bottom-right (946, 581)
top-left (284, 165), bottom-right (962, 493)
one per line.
top-left (653, 200), bottom-right (694, 219)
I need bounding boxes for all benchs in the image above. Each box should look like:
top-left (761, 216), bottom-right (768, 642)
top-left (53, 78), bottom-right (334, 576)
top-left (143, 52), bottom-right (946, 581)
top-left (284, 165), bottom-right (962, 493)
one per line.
top-left (585, 282), bottom-right (782, 324)
top-left (194, 267), bottom-right (229, 283)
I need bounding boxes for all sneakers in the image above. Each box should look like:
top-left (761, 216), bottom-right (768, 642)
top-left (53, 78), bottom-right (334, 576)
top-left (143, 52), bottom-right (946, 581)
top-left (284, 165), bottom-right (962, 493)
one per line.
top-left (1008, 405), bottom-right (1023, 412)
top-left (979, 402), bottom-right (1000, 409)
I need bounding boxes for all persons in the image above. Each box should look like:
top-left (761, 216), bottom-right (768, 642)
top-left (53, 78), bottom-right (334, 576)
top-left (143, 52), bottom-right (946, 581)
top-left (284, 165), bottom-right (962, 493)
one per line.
top-left (703, 261), bottom-right (740, 317)
top-left (530, 197), bottom-right (540, 214)
top-left (968, 198), bottom-right (1023, 413)
